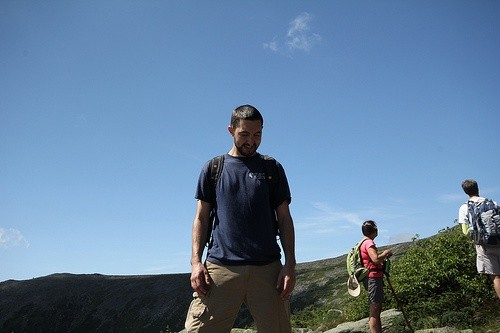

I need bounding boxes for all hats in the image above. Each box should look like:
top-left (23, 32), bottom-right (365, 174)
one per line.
top-left (347, 274), bottom-right (361, 297)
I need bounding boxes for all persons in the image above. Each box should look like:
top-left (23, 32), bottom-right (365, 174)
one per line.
top-left (184, 104), bottom-right (297, 333)
top-left (360, 220), bottom-right (392, 333)
top-left (458, 179), bottom-right (500, 298)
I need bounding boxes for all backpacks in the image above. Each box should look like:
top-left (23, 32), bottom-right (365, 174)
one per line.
top-left (463, 198), bottom-right (500, 246)
top-left (347, 237), bottom-right (372, 282)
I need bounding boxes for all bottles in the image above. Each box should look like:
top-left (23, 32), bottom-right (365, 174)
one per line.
top-left (383, 256), bottom-right (390, 274)
